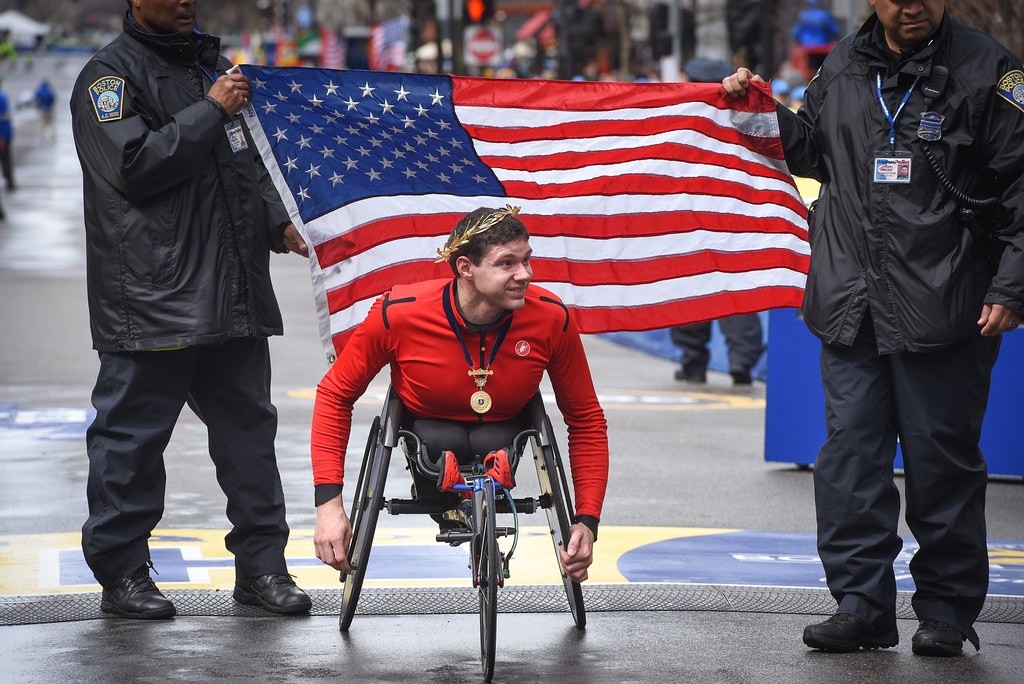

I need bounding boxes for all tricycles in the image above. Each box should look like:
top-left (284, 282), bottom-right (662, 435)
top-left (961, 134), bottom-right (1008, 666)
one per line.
top-left (335, 368), bottom-right (587, 682)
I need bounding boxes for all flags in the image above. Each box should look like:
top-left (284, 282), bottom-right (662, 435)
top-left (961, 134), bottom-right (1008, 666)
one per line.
top-left (213, 15), bottom-right (413, 71)
top-left (226, 61), bottom-right (811, 365)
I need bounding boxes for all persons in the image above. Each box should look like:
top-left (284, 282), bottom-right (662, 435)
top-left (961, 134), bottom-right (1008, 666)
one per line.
top-left (69, 1), bottom-right (314, 619)
top-left (412, 1), bottom-right (844, 114)
top-left (0, 26), bottom-right (60, 220)
top-left (721, 2), bottom-right (1023, 654)
top-left (311, 203), bottom-right (609, 586)
top-left (667, 55), bottom-right (767, 386)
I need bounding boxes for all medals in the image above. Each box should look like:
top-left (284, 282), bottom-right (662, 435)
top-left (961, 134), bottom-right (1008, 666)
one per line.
top-left (467, 364), bottom-right (494, 414)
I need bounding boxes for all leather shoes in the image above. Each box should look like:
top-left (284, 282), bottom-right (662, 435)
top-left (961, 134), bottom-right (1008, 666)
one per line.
top-left (804, 612), bottom-right (899, 651)
top-left (101, 568), bottom-right (177, 618)
top-left (912, 618), bottom-right (964, 656)
top-left (728, 365), bottom-right (751, 384)
top-left (233, 572), bottom-right (312, 613)
top-left (675, 368), bottom-right (708, 386)
